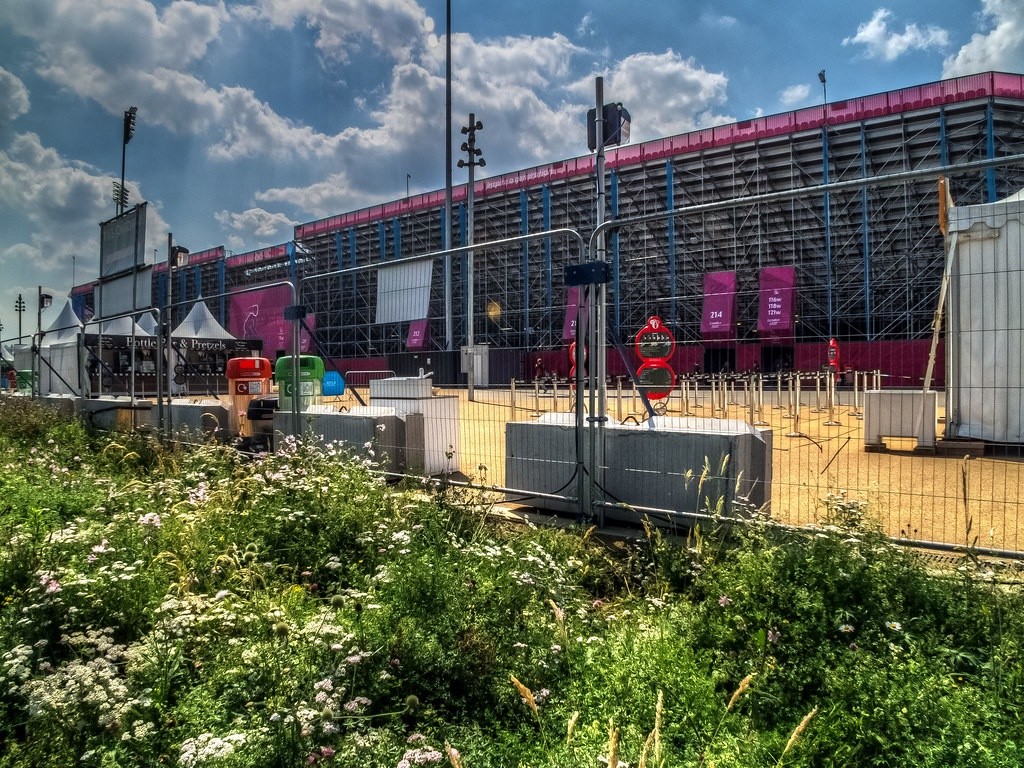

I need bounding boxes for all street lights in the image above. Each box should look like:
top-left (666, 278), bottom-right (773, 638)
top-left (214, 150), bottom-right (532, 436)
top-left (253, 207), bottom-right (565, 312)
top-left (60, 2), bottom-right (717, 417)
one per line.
top-left (587, 103), bottom-right (632, 505)
top-left (120, 106), bottom-right (138, 214)
top-left (166, 232), bottom-right (189, 403)
top-left (37, 286), bottom-right (53, 395)
top-left (456, 111), bottom-right (486, 402)
top-left (15, 294), bottom-right (25, 345)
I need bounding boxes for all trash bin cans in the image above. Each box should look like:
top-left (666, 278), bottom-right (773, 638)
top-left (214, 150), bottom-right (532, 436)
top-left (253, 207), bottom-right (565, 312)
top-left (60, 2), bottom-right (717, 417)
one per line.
top-left (225, 356), bottom-right (272, 453)
top-left (274, 355), bottom-right (325, 411)
top-left (15, 369), bottom-right (39, 392)
top-left (247, 394), bottom-right (279, 453)
top-left (8, 371), bottom-right (18, 394)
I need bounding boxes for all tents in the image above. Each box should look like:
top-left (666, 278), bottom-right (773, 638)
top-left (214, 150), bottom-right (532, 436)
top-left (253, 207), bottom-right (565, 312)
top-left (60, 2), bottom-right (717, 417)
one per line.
top-left (19, 294), bottom-right (264, 398)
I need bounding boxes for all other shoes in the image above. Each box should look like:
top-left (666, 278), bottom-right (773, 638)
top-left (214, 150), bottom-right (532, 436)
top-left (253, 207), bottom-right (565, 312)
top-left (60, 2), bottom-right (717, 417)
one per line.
top-left (544, 388), bottom-right (547, 394)
